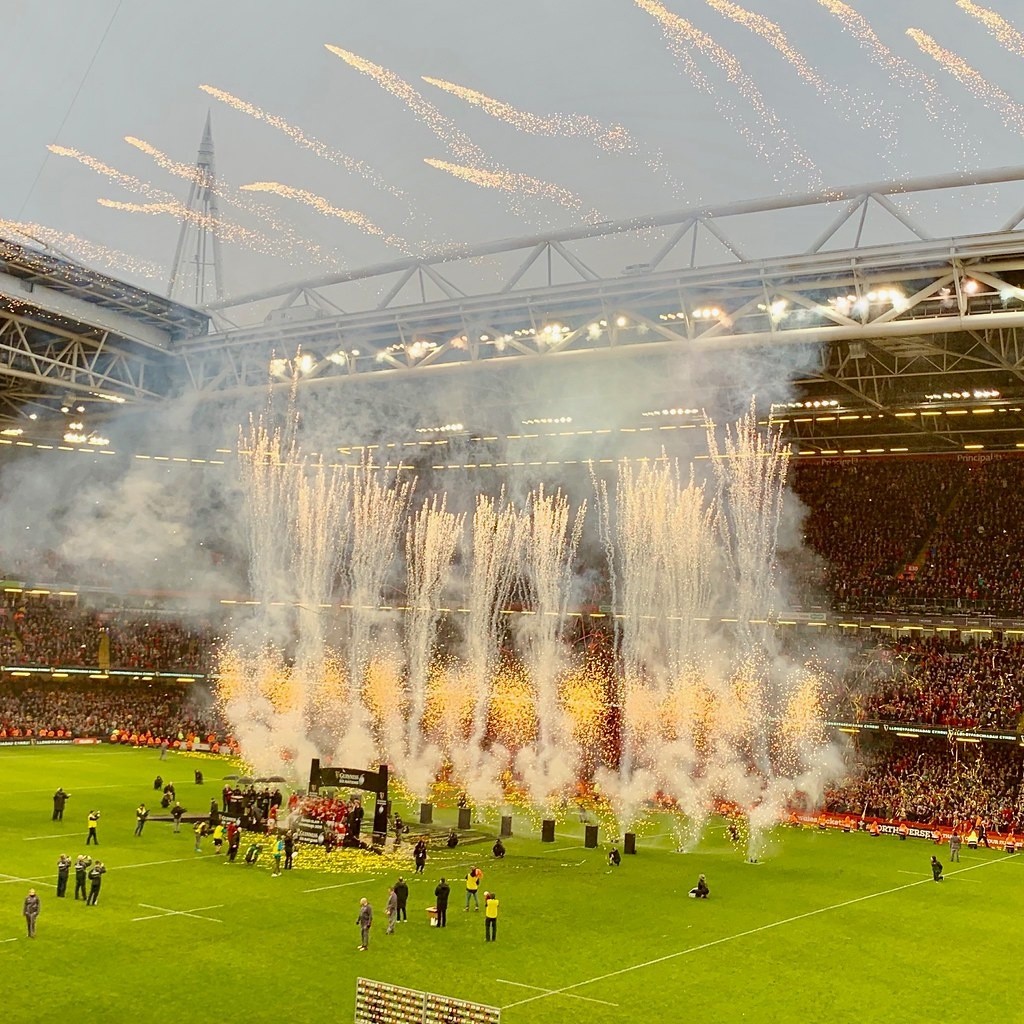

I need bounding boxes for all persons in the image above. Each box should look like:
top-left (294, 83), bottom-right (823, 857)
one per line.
top-left (386, 876), bottom-right (409, 935)
top-left (134, 803), bottom-right (150, 837)
top-left (194, 769), bottom-right (203, 785)
top-left (24, 888), bottom-right (40, 939)
top-left (53, 786), bottom-right (71, 821)
top-left (57, 854), bottom-right (107, 906)
top-left (86, 809), bottom-right (100, 846)
top-left (462, 865), bottom-right (499, 942)
top-left (0, 459), bottom-right (1024, 757)
top-left (171, 802), bottom-right (186, 833)
top-left (695, 874), bottom-right (710, 899)
top-left (189, 785), bottom-right (506, 876)
top-left (153, 775), bottom-right (175, 808)
top-left (357, 898), bottom-right (373, 952)
top-left (434, 877), bottom-right (449, 927)
top-left (507, 751), bottom-right (1023, 882)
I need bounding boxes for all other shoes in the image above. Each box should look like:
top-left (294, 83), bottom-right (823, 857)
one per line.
top-left (358, 945), bottom-right (363, 949)
top-left (404, 920), bottom-right (407, 923)
top-left (359, 947), bottom-right (367, 951)
top-left (396, 920), bottom-right (400, 923)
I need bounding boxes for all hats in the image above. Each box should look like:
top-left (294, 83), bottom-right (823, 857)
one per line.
top-left (699, 874), bottom-right (706, 881)
